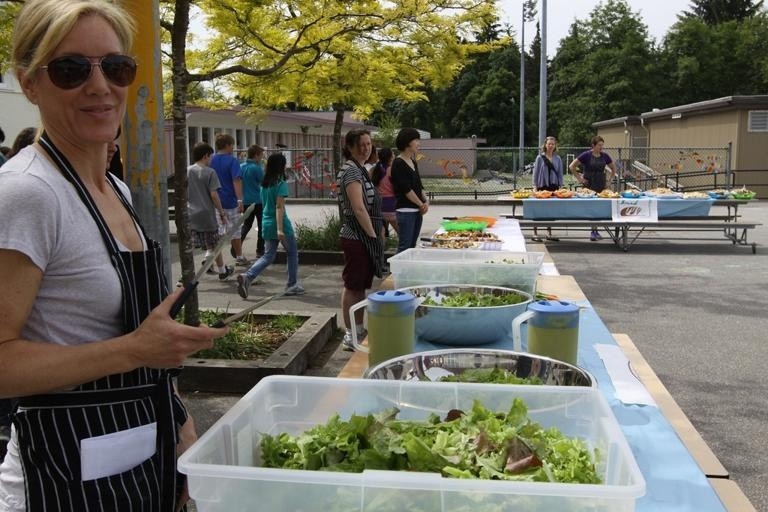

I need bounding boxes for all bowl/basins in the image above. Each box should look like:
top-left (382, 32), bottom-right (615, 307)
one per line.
top-left (710, 190), bottom-right (758, 199)
top-left (441, 215), bottom-right (497, 231)
top-left (364, 348), bottom-right (596, 427)
top-left (511, 191), bottom-right (640, 199)
top-left (398, 282), bottom-right (532, 347)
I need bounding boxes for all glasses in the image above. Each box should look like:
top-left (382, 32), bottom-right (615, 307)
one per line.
top-left (40, 53), bottom-right (138, 90)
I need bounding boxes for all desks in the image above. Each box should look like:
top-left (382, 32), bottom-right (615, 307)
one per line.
top-left (498, 195), bottom-right (759, 249)
top-left (295, 331), bottom-right (733, 479)
top-left (708, 475), bottom-right (756, 511)
top-left (527, 241), bottom-right (559, 275)
top-left (367, 274), bottom-right (607, 332)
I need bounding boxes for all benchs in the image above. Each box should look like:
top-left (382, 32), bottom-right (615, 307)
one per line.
top-left (500, 213), bottom-right (742, 235)
top-left (515, 220), bottom-right (763, 254)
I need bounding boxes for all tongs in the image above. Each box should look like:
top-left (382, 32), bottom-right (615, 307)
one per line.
top-left (170, 202), bottom-right (315, 329)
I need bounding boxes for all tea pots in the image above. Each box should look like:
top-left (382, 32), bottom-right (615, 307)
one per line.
top-left (512, 300), bottom-right (586, 364)
top-left (350, 289), bottom-right (428, 370)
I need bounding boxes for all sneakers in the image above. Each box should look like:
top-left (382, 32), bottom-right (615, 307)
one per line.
top-left (589, 230), bottom-right (601, 241)
top-left (283, 285), bottom-right (307, 297)
top-left (342, 328), bottom-right (368, 352)
top-left (236, 257), bottom-right (251, 266)
top-left (532, 233), bottom-right (559, 242)
top-left (218, 264), bottom-right (234, 281)
top-left (236, 272), bottom-right (251, 299)
top-left (201, 259), bottom-right (213, 274)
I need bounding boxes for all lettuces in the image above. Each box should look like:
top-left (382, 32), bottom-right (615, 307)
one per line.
top-left (420, 294), bottom-right (522, 307)
top-left (441, 367), bottom-right (545, 384)
top-left (256, 396), bottom-right (606, 512)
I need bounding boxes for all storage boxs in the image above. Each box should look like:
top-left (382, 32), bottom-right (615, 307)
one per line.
top-left (385, 245), bottom-right (545, 295)
top-left (173, 374), bottom-right (648, 512)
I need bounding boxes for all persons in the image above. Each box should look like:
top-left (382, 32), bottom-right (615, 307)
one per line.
top-left (0, 127), bottom-right (38, 168)
top-left (103, 127), bottom-right (122, 171)
top-left (176, 133), bottom-right (304, 298)
top-left (335, 128), bottom-right (428, 348)
top-left (568, 136), bottom-right (614, 239)
top-left (532, 137), bottom-right (563, 242)
top-left (0, 0), bottom-right (230, 511)
